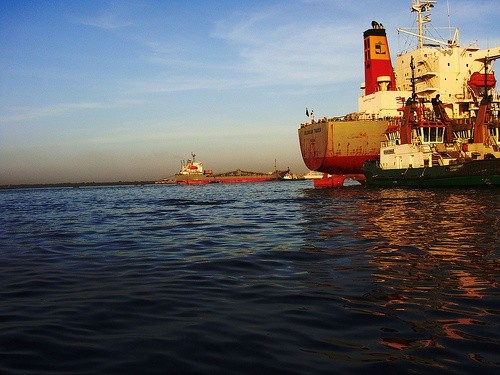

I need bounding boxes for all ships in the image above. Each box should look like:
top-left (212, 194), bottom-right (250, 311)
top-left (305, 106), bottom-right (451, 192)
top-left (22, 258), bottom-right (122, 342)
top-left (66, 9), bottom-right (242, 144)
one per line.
top-left (173, 153), bottom-right (289, 185)
top-left (297, 0), bottom-right (500, 190)
